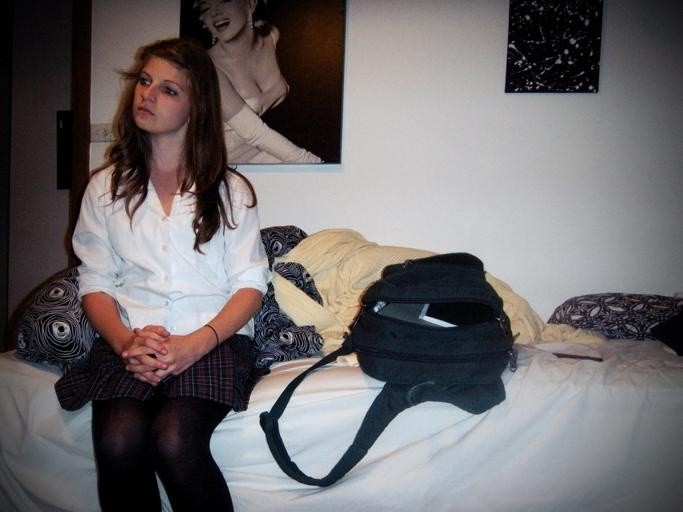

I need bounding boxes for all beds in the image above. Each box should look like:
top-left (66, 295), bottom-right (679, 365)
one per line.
top-left (0, 337), bottom-right (683, 512)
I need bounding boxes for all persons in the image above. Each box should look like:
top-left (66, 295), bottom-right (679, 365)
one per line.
top-left (195, 0), bottom-right (325, 164)
top-left (55, 38), bottom-right (272, 512)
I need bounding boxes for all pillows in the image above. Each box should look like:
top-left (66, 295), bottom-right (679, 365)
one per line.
top-left (545, 295), bottom-right (679, 349)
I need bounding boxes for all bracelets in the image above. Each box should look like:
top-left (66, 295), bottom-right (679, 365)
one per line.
top-left (204, 325), bottom-right (219, 347)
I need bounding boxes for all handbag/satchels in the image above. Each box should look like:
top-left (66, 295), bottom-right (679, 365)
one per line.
top-left (354, 252), bottom-right (515, 389)
